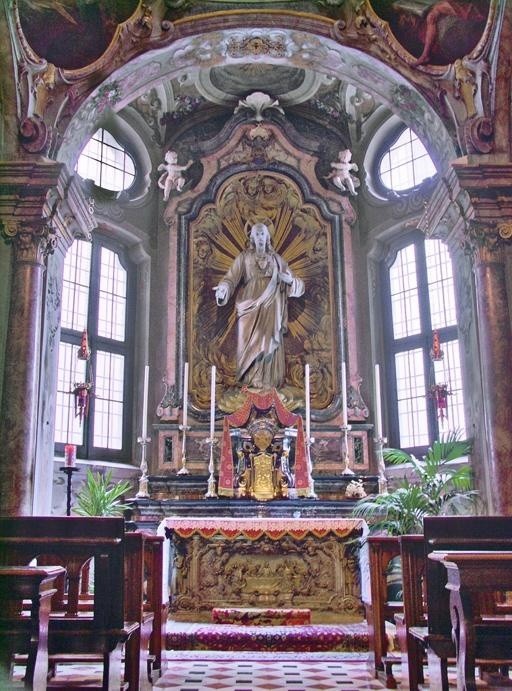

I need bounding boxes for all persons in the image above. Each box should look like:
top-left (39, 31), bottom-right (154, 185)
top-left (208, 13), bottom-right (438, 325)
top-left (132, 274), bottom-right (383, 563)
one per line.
top-left (329, 149), bottom-right (361, 196)
top-left (211, 222), bottom-right (308, 387)
top-left (238, 419), bottom-right (282, 502)
top-left (156, 151), bottom-right (194, 204)
top-left (411, 1), bottom-right (483, 68)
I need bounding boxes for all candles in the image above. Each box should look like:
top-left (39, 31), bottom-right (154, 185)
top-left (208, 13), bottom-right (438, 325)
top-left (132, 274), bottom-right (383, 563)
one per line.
top-left (141, 361), bottom-right (383, 442)
top-left (64, 443), bottom-right (77, 466)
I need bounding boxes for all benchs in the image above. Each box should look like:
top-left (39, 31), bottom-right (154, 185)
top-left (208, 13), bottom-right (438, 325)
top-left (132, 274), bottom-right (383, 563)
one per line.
top-left (211, 607), bottom-right (311, 625)
top-left (367, 515), bottom-right (511, 691)
top-left (0, 515), bottom-right (170, 691)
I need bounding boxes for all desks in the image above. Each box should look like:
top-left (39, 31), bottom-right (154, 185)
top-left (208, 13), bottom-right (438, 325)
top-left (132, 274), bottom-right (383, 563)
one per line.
top-left (164, 516), bottom-right (365, 626)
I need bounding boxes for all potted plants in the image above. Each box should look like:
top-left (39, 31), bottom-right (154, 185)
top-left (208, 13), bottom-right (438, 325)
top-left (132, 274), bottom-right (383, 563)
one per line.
top-left (353, 424), bottom-right (492, 653)
